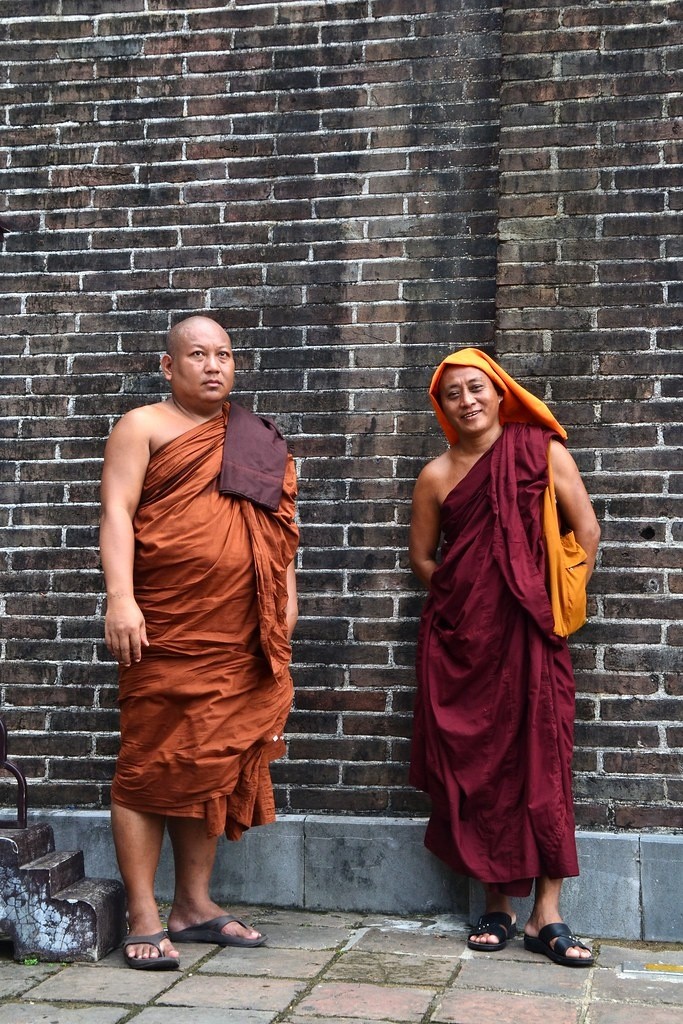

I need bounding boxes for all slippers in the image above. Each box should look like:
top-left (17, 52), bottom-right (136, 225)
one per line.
top-left (167, 914), bottom-right (269, 948)
top-left (467, 911), bottom-right (516, 951)
top-left (523, 922), bottom-right (594, 965)
top-left (121, 929), bottom-right (179, 969)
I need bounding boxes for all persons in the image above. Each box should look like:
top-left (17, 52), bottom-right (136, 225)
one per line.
top-left (409, 348), bottom-right (603, 967)
top-left (99, 316), bottom-right (302, 970)
